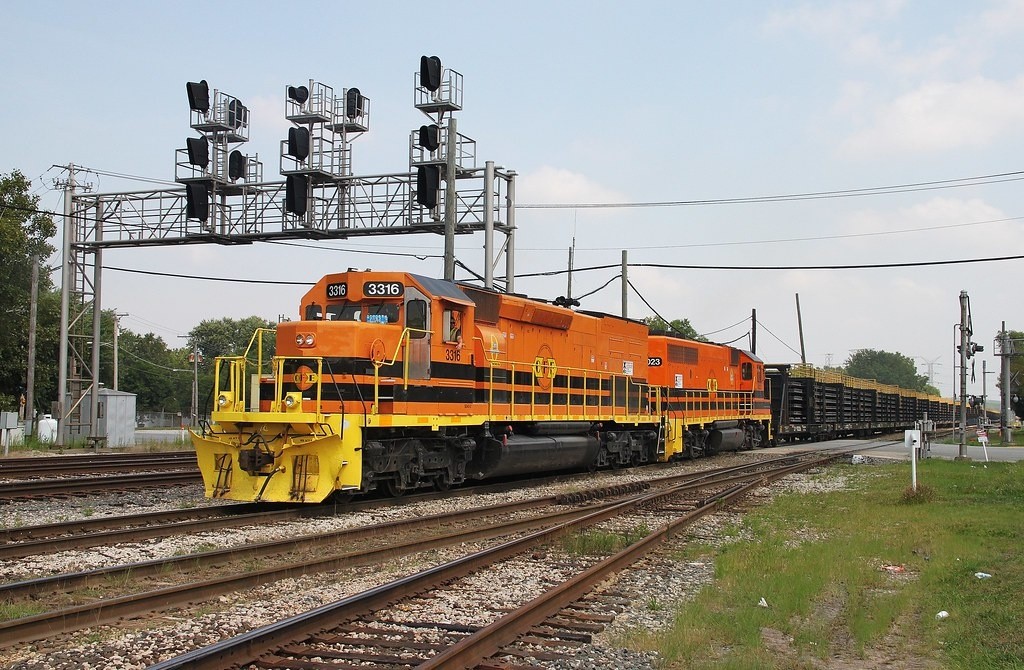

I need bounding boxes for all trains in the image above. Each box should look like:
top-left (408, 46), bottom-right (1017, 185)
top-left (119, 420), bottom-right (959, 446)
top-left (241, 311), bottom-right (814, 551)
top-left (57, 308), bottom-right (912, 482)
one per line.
top-left (190, 268), bottom-right (999, 504)
